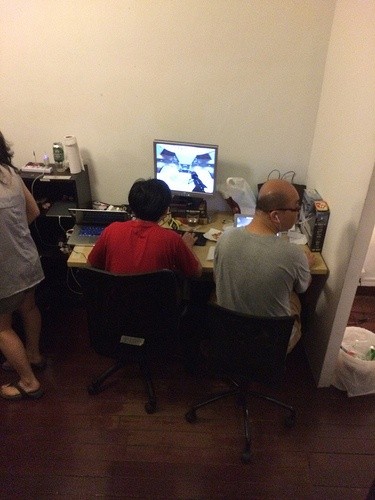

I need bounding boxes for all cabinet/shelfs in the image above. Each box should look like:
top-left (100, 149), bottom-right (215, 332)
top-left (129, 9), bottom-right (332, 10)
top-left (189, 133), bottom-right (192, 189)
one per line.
top-left (20, 164), bottom-right (94, 307)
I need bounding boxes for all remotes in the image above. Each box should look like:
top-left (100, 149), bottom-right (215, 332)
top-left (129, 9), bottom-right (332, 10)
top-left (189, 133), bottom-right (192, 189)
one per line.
top-left (152, 139), bottom-right (218, 205)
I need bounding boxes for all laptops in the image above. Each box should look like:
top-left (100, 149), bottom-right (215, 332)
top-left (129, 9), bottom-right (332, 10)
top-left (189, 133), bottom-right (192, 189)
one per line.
top-left (67, 208), bottom-right (132, 246)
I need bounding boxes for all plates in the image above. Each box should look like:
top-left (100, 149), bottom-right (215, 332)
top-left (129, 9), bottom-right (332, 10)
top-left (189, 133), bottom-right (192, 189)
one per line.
top-left (203, 228), bottom-right (224, 242)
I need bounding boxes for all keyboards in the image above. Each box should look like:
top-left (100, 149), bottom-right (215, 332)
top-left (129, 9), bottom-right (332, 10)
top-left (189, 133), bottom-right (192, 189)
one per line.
top-left (173, 230), bottom-right (207, 245)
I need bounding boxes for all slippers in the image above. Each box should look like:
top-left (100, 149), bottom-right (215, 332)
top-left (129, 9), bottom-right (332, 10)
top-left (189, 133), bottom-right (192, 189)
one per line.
top-left (0, 357), bottom-right (47, 372)
top-left (0, 381), bottom-right (43, 400)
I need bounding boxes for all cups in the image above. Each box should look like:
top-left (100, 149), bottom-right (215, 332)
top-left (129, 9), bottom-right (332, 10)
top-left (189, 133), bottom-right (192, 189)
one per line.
top-left (53, 143), bottom-right (65, 173)
top-left (186, 210), bottom-right (200, 226)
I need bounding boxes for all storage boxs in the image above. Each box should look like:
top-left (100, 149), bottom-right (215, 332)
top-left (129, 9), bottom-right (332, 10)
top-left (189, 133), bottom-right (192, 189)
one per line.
top-left (299, 189), bottom-right (329, 252)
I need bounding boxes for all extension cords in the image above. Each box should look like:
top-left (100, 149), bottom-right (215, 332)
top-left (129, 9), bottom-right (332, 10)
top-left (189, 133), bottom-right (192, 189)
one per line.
top-left (21, 166), bottom-right (54, 173)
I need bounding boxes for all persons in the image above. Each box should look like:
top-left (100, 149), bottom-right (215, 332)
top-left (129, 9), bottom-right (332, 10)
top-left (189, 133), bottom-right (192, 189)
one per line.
top-left (0, 130), bottom-right (46, 399)
top-left (85, 178), bottom-right (202, 277)
top-left (214, 181), bottom-right (312, 357)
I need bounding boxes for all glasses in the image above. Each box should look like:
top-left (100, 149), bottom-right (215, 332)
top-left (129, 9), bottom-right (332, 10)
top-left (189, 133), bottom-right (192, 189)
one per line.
top-left (270, 205), bottom-right (302, 213)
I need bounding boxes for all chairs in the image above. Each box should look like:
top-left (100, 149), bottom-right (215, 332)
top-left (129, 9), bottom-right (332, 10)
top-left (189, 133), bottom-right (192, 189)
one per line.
top-left (77, 269), bottom-right (304, 465)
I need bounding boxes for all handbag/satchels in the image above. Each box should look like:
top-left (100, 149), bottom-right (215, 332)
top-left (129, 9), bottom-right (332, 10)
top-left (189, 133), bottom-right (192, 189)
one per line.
top-left (257, 170), bottom-right (306, 206)
top-left (216, 176), bottom-right (257, 216)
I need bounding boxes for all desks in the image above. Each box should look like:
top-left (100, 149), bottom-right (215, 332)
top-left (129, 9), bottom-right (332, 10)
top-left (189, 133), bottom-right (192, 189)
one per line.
top-left (66, 212), bottom-right (329, 346)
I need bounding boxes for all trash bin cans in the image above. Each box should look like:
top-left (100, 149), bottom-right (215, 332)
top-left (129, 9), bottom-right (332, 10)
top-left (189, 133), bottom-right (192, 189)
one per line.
top-left (331, 327), bottom-right (375, 391)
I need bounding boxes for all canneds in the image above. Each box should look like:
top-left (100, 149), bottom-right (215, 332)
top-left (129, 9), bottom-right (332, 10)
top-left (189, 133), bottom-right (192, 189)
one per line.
top-left (52, 142), bottom-right (65, 163)
